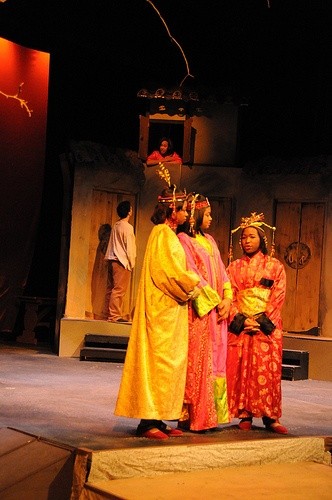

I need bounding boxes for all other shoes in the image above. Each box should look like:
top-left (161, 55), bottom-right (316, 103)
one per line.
top-left (239, 419), bottom-right (251, 431)
top-left (136, 424), bottom-right (167, 438)
top-left (111, 318), bottom-right (132, 324)
top-left (265, 423), bottom-right (288, 434)
top-left (158, 421), bottom-right (183, 436)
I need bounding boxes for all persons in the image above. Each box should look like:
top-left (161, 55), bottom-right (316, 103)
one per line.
top-left (225, 212), bottom-right (291, 435)
top-left (104, 199), bottom-right (138, 325)
top-left (111, 183), bottom-right (201, 439)
top-left (145, 140), bottom-right (182, 168)
top-left (178, 194), bottom-right (232, 431)
